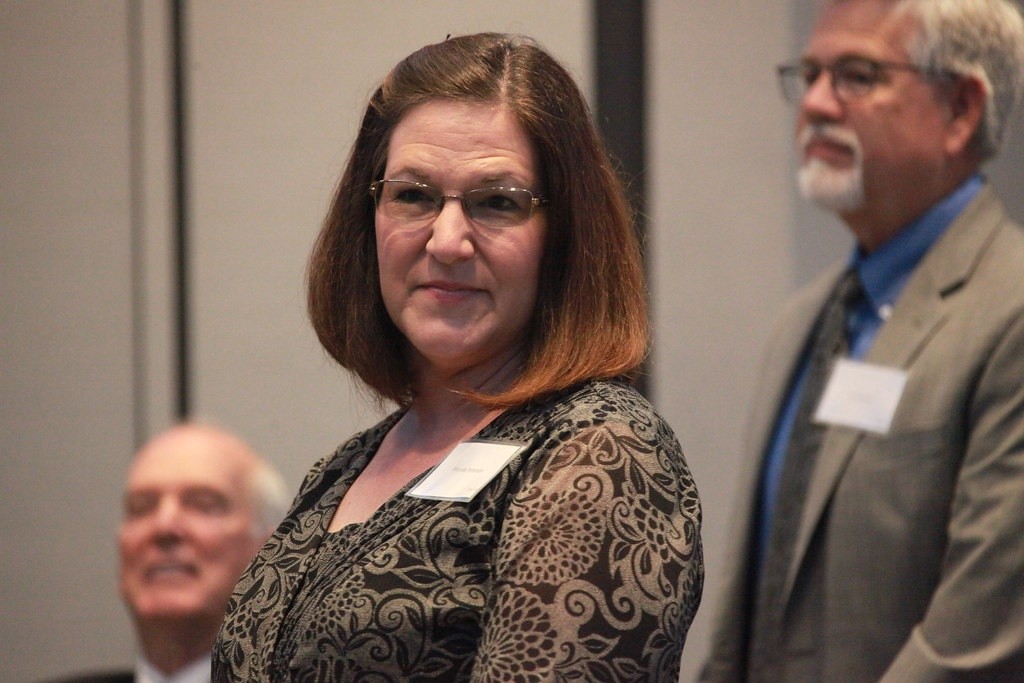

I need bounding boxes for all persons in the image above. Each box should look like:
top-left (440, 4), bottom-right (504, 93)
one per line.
top-left (36, 419), bottom-right (290, 682)
top-left (696, 1), bottom-right (1023, 682)
top-left (206, 28), bottom-right (706, 683)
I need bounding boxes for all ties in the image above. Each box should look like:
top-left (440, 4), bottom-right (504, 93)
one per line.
top-left (763, 269), bottom-right (861, 647)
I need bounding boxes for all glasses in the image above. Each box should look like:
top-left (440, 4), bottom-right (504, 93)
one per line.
top-left (775, 55), bottom-right (940, 104)
top-left (369, 179), bottom-right (550, 226)
